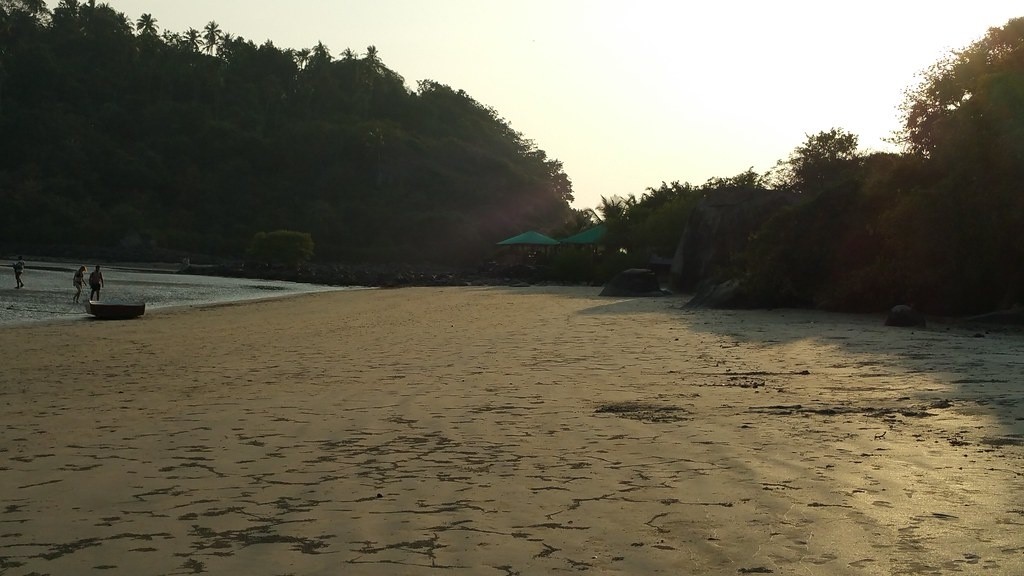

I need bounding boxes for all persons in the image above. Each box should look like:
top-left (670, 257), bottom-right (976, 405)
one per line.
top-left (88, 266), bottom-right (104, 301)
top-left (14, 255), bottom-right (24, 289)
top-left (72, 266), bottom-right (87, 303)
